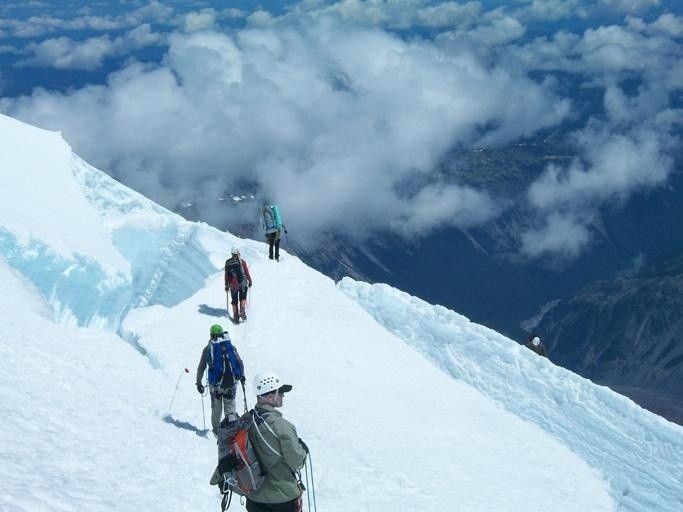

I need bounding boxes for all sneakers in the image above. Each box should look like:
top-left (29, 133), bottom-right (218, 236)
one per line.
top-left (233, 313), bottom-right (247, 324)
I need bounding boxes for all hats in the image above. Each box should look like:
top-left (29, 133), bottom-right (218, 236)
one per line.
top-left (256, 375), bottom-right (292, 396)
top-left (231, 246), bottom-right (241, 255)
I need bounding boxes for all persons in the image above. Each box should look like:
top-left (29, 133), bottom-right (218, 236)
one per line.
top-left (224, 246), bottom-right (253, 321)
top-left (264, 224), bottom-right (288, 260)
top-left (196, 324), bottom-right (245, 435)
top-left (527, 337), bottom-right (547, 357)
top-left (240, 372), bottom-right (308, 511)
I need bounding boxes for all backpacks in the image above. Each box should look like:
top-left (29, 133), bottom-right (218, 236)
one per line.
top-left (210, 409), bottom-right (284, 497)
top-left (261, 206), bottom-right (277, 232)
top-left (208, 338), bottom-right (241, 388)
top-left (225, 258), bottom-right (247, 290)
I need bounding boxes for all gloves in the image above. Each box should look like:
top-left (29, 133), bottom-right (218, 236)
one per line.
top-left (197, 383), bottom-right (205, 394)
top-left (240, 376), bottom-right (246, 385)
top-left (225, 287), bottom-right (229, 292)
top-left (248, 281), bottom-right (253, 288)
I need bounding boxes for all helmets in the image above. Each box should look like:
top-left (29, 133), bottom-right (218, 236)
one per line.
top-left (210, 324), bottom-right (224, 336)
top-left (532, 337), bottom-right (541, 346)
